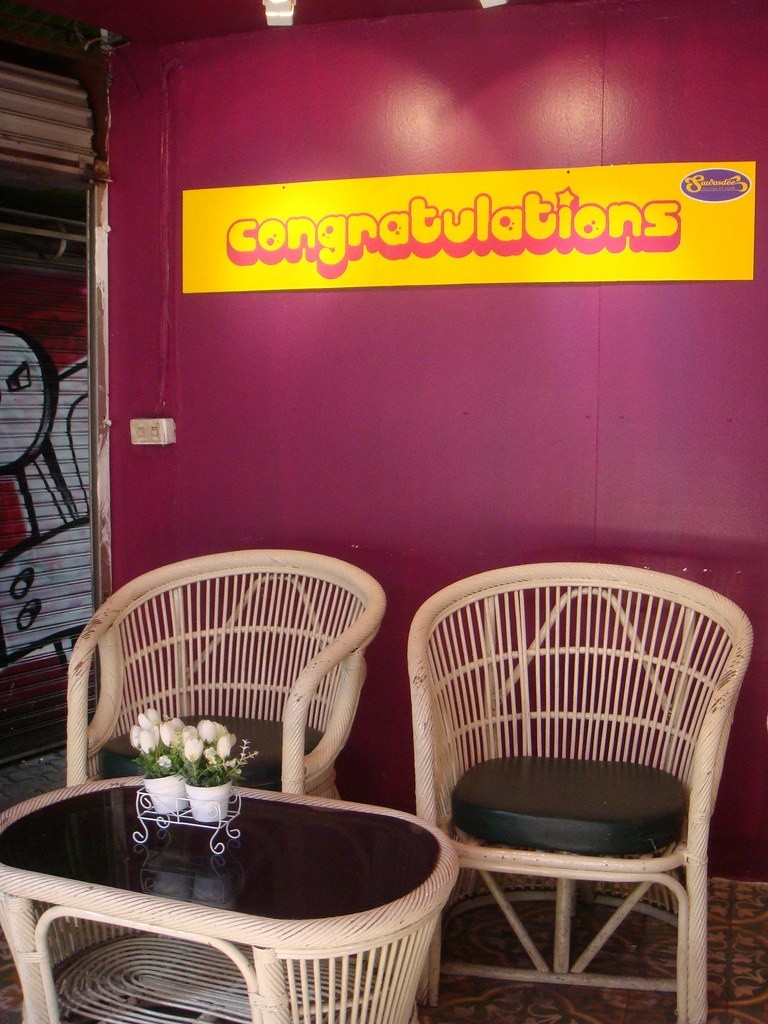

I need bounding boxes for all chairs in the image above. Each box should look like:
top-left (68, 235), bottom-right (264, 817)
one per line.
top-left (403, 561), bottom-right (755, 1024)
top-left (66, 547), bottom-right (386, 799)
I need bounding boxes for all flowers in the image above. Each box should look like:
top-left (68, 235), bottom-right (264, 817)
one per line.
top-left (180, 720), bottom-right (258, 788)
top-left (128, 707), bottom-right (186, 779)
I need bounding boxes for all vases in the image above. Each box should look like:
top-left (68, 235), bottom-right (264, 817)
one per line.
top-left (185, 779), bottom-right (234, 824)
top-left (143, 776), bottom-right (189, 815)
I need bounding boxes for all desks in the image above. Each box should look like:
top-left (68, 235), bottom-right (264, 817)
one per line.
top-left (0, 776), bottom-right (460, 1024)
